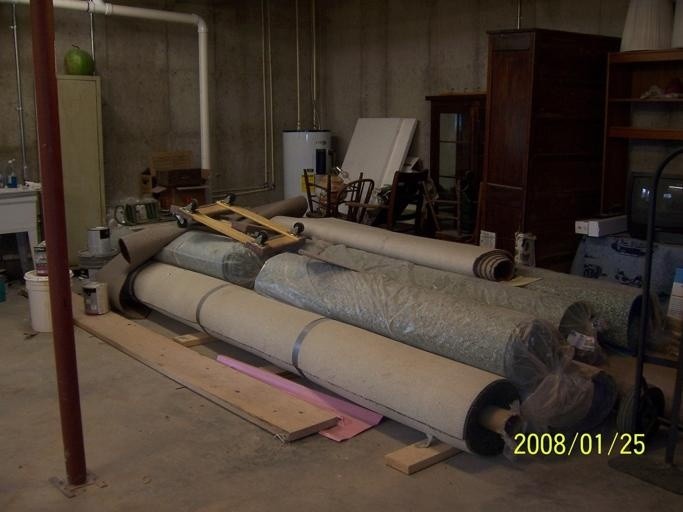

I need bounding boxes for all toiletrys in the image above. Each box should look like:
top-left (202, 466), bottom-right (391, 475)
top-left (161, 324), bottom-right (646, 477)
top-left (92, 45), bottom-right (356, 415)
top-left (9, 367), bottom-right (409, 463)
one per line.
top-left (6, 158), bottom-right (18, 187)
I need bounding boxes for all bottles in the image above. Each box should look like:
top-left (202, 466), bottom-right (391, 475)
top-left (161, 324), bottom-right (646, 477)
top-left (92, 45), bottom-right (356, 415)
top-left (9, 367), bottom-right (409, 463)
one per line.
top-left (7, 160), bottom-right (16, 187)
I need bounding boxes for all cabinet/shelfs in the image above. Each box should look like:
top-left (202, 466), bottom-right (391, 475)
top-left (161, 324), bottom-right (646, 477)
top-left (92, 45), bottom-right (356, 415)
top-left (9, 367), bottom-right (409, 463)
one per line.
top-left (55, 73), bottom-right (107, 273)
top-left (484, 26), bottom-right (622, 272)
top-left (599, 49), bottom-right (682, 213)
top-left (425, 94), bottom-right (485, 200)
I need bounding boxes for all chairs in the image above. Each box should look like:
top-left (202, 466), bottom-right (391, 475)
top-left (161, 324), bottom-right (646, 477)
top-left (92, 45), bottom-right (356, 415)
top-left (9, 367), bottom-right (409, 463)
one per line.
top-left (301, 168), bottom-right (483, 246)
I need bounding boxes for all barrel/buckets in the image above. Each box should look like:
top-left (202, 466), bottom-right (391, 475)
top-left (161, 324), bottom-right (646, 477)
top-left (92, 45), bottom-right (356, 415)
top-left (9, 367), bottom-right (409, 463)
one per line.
top-left (24, 269), bottom-right (73, 333)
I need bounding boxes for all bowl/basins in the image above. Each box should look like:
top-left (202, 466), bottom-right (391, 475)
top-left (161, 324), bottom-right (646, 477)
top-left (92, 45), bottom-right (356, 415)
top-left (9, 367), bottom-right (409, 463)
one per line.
top-left (124, 202), bottom-right (160, 224)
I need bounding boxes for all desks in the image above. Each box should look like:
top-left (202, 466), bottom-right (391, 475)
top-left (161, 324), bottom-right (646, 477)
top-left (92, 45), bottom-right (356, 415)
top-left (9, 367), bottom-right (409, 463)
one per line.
top-left (0, 188), bottom-right (40, 271)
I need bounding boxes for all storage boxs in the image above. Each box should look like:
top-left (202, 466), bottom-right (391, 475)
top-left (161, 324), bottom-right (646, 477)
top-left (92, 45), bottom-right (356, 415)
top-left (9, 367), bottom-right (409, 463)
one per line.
top-left (140, 167), bottom-right (207, 211)
